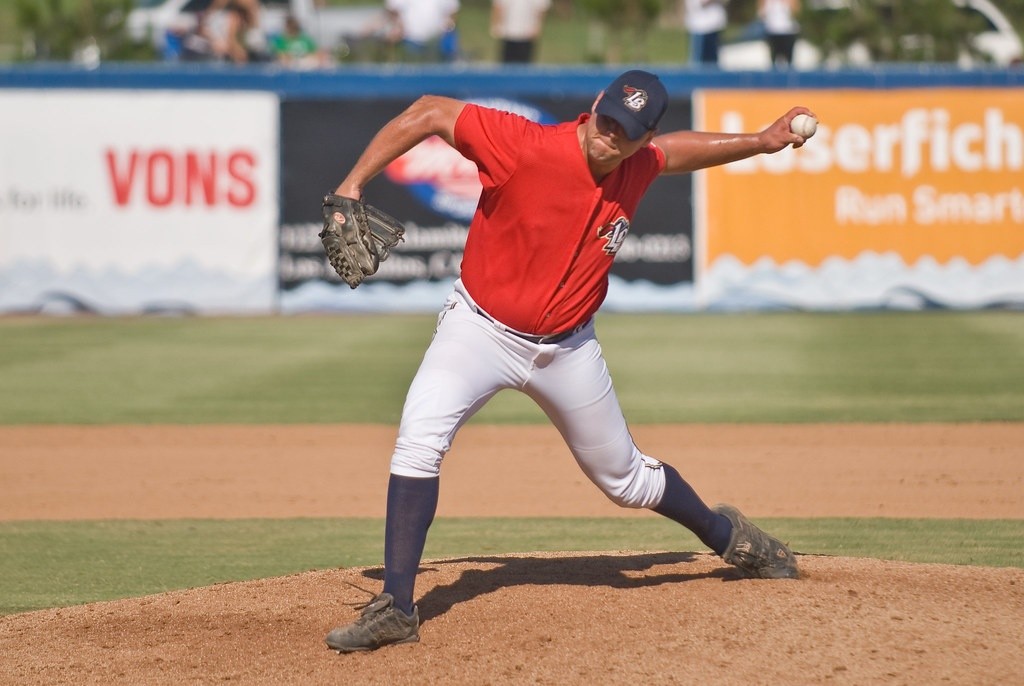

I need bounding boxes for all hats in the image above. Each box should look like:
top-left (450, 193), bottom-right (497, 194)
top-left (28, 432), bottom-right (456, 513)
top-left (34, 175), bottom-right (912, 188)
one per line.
top-left (594, 70), bottom-right (669, 141)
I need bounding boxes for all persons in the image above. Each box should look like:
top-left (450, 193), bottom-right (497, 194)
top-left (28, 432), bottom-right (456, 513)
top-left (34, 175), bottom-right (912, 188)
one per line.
top-left (490, 0), bottom-right (553, 65)
top-left (683, 0), bottom-right (727, 64)
top-left (758, 0), bottom-right (801, 70)
top-left (161, 0), bottom-right (462, 68)
top-left (320, 68), bottom-right (821, 653)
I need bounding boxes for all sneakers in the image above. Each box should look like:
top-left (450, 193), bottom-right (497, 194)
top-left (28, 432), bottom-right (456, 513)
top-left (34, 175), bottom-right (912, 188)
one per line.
top-left (710, 503), bottom-right (801, 580)
top-left (326, 593), bottom-right (419, 651)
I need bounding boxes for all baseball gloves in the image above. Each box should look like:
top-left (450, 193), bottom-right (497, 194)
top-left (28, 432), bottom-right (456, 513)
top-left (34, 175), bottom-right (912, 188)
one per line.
top-left (316, 187), bottom-right (409, 294)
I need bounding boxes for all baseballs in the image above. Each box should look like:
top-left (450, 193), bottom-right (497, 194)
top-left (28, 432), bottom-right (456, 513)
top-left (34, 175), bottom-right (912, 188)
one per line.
top-left (790, 113), bottom-right (818, 139)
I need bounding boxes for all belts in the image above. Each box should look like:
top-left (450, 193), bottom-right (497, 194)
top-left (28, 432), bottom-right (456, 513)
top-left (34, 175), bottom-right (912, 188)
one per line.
top-left (477, 309), bottom-right (592, 343)
